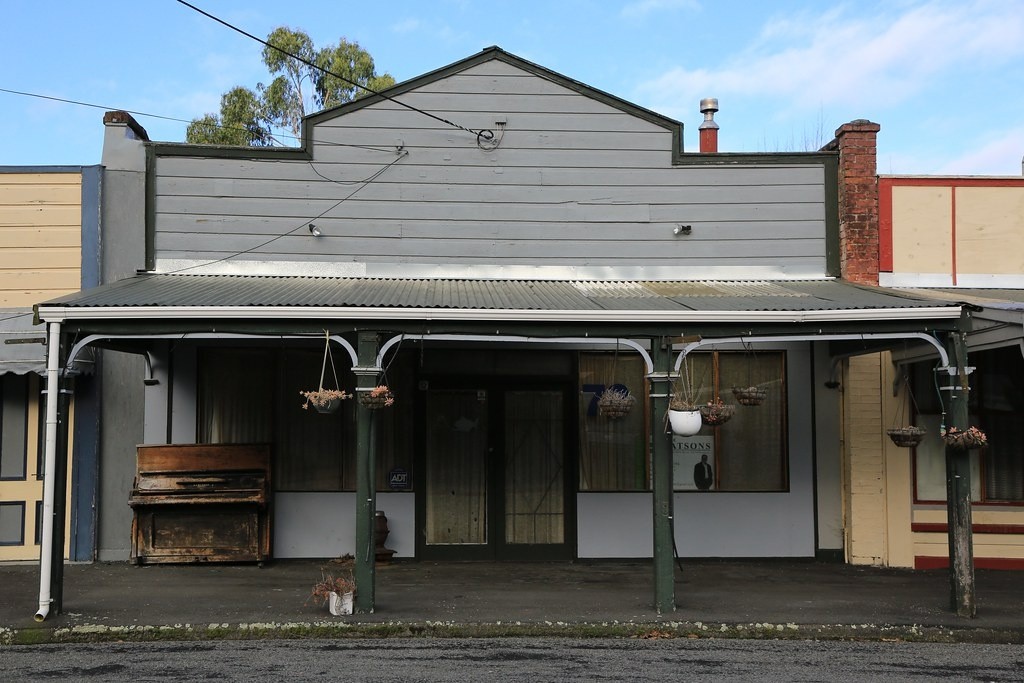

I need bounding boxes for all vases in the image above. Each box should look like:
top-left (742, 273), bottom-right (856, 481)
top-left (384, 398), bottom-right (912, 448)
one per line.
top-left (313, 397), bottom-right (341, 414)
top-left (327, 591), bottom-right (354, 617)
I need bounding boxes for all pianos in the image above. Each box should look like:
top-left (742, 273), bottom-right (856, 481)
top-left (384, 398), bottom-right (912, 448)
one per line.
top-left (127, 441), bottom-right (273, 569)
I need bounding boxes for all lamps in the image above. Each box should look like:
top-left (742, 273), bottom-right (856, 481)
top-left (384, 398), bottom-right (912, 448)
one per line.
top-left (308, 223), bottom-right (322, 237)
top-left (673, 223), bottom-right (692, 235)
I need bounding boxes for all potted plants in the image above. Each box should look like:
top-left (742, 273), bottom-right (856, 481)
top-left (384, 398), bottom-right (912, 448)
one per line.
top-left (593, 387), bottom-right (637, 418)
top-left (664, 372), bottom-right (703, 437)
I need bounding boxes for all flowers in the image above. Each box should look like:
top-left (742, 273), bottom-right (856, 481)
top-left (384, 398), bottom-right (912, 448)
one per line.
top-left (301, 575), bottom-right (357, 614)
top-left (299, 388), bottom-right (354, 410)
top-left (705, 398), bottom-right (725, 422)
top-left (370, 384), bottom-right (394, 407)
top-left (940, 426), bottom-right (987, 450)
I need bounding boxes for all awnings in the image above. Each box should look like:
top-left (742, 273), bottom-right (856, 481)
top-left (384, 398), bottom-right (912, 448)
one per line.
top-left (0, 307), bottom-right (96, 378)
